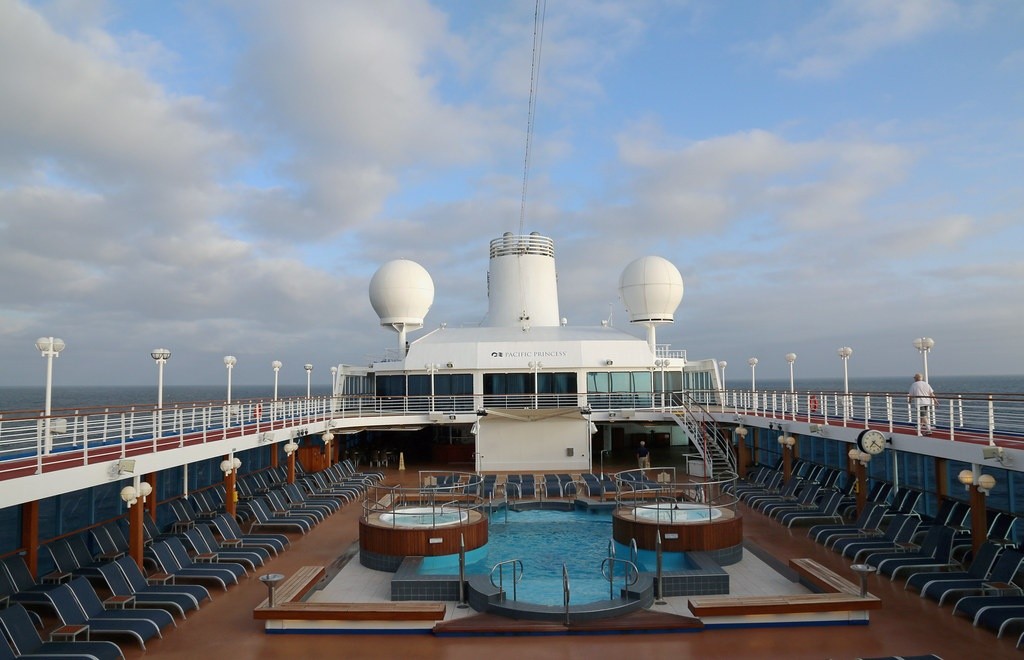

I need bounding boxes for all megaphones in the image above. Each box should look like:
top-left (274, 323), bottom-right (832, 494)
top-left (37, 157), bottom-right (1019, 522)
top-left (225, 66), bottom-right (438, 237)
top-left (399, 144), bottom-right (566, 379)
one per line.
top-left (449, 414), bottom-right (456, 420)
top-left (608, 411), bottom-right (616, 417)
top-left (607, 359), bottom-right (613, 365)
top-left (447, 361), bottom-right (454, 367)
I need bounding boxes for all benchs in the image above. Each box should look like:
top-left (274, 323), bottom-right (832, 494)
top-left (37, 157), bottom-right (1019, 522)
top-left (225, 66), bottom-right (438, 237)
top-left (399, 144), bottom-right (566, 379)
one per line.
top-left (603, 488), bottom-right (717, 507)
top-left (370, 494), bottom-right (478, 511)
top-left (689, 556), bottom-right (883, 617)
top-left (251, 566), bottom-right (447, 621)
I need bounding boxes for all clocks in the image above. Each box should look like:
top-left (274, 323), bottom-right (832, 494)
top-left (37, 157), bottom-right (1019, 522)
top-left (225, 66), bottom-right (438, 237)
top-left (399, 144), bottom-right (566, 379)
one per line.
top-left (857, 428), bottom-right (893, 455)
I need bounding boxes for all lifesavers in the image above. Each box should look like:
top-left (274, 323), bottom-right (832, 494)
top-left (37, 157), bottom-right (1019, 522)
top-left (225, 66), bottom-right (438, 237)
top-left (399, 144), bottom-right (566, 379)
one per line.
top-left (809, 396), bottom-right (817, 412)
top-left (254, 403), bottom-right (262, 418)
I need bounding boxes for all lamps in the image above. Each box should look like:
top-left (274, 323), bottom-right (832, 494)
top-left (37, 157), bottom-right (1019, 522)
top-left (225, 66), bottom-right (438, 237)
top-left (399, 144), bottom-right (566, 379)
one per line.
top-left (984, 446), bottom-right (1013, 469)
top-left (120, 482), bottom-right (138, 509)
top-left (322, 433), bottom-right (335, 445)
top-left (140, 482), bottom-right (153, 503)
top-left (118, 460), bottom-right (135, 475)
top-left (233, 458), bottom-right (242, 474)
top-left (736, 427), bottom-right (747, 440)
top-left (957, 469), bottom-right (974, 492)
top-left (262, 432), bottom-right (274, 442)
top-left (849, 449), bottom-right (871, 468)
top-left (977, 474), bottom-right (997, 497)
top-left (778, 435), bottom-right (796, 450)
top-left (809, 424), bottom-right (832, 437)
top-left (732, 414), bottom-right (744, 423)
top-left (219, 460), bottom-right (233, 477)
top-left (284, 442), bottom-right (299, 457)
top-left (328, 418), bottom-right (338, 428)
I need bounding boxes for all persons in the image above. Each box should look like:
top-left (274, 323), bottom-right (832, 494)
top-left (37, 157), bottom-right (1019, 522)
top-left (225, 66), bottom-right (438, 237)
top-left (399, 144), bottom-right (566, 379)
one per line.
top-left (636, 441), bottom-right (650, 475)
top-left (907, 373), bottom-right (941, 436)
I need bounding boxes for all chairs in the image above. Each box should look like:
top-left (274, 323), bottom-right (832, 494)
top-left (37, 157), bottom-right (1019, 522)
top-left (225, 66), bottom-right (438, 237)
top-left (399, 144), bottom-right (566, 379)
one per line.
top-left (718, 453), bottom-right (1024, 660)
top-left (0, 446), bottom-right (399, 660)
top-left (420, 469), bottom-right (688, 505)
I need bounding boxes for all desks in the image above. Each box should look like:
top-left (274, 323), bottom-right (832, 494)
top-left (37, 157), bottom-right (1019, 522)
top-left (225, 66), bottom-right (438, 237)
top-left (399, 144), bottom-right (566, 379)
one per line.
top-left (345, 451), bottom-right (392, 468)
